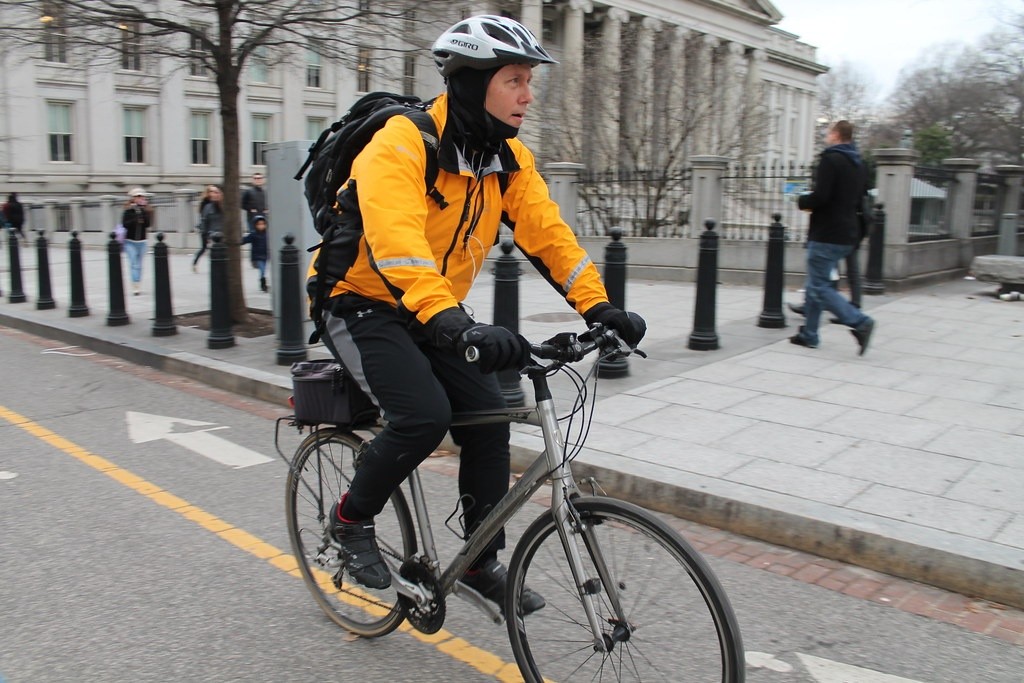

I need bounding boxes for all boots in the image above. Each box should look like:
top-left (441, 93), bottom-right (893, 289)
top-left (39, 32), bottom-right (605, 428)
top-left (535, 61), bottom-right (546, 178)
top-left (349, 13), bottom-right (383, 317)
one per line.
top-left (261, 277), bottom-right (268, 291)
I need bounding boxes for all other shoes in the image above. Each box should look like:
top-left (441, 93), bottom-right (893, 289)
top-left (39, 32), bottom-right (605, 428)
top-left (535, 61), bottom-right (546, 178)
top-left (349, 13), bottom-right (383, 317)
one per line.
top-left (192, 264), bottom-right (197, 272)
top-left (790, 330), bottom-right (818, 348)
top-left (851, 318), bottom-right (874, 355)
top-left (133, 283), bottom-right (140, 294)
top-left (788, 304), bottom-right (806, 317)
top-left (829, 317), bottom-right (844, 324)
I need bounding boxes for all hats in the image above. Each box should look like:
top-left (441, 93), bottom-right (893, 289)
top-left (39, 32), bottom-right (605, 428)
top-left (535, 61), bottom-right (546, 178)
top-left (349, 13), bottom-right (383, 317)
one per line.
top-left (131, 189), bottom-right (145, 196)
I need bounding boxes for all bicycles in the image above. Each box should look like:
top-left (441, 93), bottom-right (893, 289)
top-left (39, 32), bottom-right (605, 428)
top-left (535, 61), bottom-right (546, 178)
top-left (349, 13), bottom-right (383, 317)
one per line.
top-left (276, 322), bottom-right (745, 683)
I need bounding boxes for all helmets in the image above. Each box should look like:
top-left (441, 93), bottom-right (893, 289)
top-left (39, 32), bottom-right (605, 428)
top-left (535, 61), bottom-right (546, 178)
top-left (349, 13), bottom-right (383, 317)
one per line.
top-left (431, 14), bottom-right (559, 78)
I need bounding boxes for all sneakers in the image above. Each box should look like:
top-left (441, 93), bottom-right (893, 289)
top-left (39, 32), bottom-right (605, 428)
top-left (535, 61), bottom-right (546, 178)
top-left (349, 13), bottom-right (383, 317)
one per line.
top-left (459, 560), bottom-right (546, 615)
top-left (330, 493), bottom-right (391, 589)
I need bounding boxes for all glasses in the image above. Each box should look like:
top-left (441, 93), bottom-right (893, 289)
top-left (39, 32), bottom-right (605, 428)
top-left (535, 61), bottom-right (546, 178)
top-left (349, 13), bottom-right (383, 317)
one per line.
top-left (254, 178), bottom-right (262, 179)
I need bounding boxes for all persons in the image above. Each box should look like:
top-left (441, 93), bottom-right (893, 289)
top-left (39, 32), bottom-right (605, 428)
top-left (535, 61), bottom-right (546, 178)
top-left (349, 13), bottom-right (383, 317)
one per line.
top-left (113, 223), bottom-right (128, 252)
top-left (240, 215), bottom-right (270, 291)
top-left (306, 15), bottom-right (646, 614)
top-left (790, 120), bottom-right (876, 356)
top-left (787, 153), bottom-right (873, 324)
top-left (193, 184), bottom-right (223, 272)
top-left (240, 172), bottom-right (270, 268)
top-left (3, 194), bottom-right (28, 247)
top-left (199, 186), bottom-right (224, 232)
top-left (122, 189), bottom-right (153, 295)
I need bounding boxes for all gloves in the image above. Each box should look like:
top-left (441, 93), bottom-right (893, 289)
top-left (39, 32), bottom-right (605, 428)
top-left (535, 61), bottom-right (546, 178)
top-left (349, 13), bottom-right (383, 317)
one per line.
top-left (431, 308), bottom-right (521, 375)
top-left (585, 302), bottom-right (647, 344)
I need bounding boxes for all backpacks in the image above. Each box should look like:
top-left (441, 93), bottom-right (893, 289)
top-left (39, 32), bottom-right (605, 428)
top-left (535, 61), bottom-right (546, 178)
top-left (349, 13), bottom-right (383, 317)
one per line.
top-left (291, 359), bottom-right (380, 424)
top-left (293, 92), bottom-right (440, 252)
